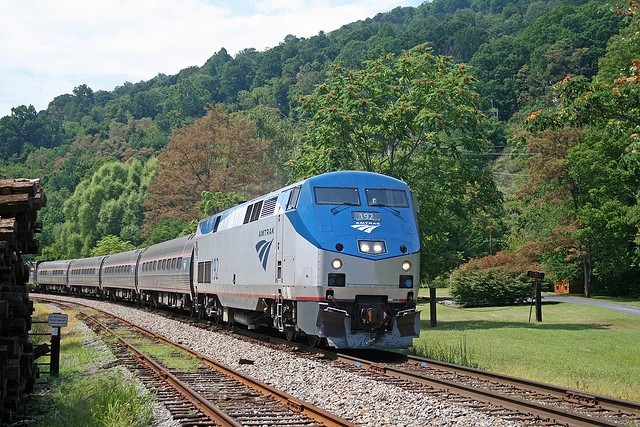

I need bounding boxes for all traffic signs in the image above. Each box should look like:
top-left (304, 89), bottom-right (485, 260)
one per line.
top-left (48, 314), bottom-right (68, 327)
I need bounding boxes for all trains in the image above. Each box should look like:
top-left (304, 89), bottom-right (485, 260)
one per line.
top-left (26, 170), bottom-right (423, 351)
